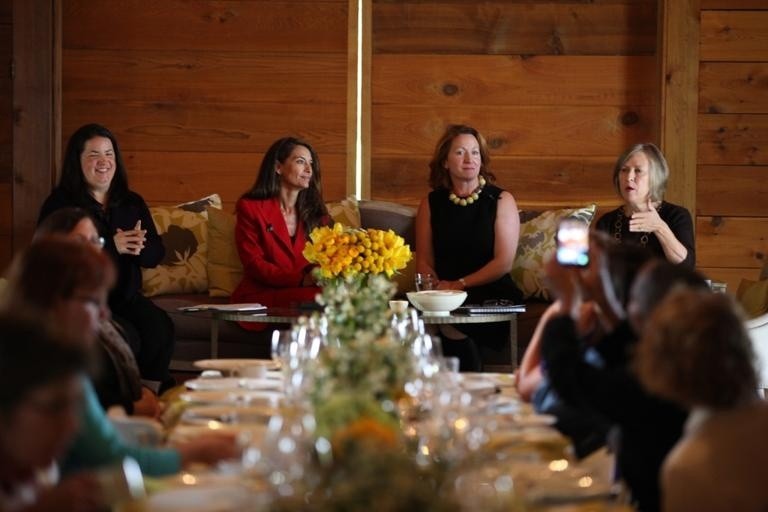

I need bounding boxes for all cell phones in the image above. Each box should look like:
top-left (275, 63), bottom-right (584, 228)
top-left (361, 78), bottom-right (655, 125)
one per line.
top-left (557, 216), bottom-right (591, 267)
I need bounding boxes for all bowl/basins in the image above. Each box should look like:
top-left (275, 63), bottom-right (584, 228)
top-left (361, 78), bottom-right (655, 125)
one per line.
top-left (388, 300), bottom-right (409, 315)
top-left (406, 289), bottom-right (468, 317)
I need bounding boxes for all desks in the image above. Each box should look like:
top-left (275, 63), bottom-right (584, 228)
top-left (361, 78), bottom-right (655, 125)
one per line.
top-left (177, 301), bottom-right (529, 375)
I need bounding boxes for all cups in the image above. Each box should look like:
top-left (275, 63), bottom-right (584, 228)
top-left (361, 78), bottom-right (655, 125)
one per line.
top-left (415, 272), bottom-right (434, 291)
top-left (243, 314), bottom-right (542, 512)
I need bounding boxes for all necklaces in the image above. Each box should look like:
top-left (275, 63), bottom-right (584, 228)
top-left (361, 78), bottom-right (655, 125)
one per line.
top-left (449, 175), bottom-right (486, 207)
top-left (615, 206), bottom-right (664, 249)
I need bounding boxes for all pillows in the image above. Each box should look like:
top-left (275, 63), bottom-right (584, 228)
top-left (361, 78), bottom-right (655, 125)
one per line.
top-left (200, 203), bottom-right (244, 299)
top-left (354, 197), bottom-right (414, 238)
top-left (136, 196), bottom-right (223, 297)
top-left (510, 205), bottom-right (603, 301)
top-left (321, 201), bottom-right (357, 222)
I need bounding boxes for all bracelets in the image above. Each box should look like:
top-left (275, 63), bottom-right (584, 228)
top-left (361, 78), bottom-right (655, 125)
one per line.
top-left (458, 276), bottom-right (467, 287)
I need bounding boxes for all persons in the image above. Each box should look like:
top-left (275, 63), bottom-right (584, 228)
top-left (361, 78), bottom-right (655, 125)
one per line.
top-left (415, 124), bottom-right (524, 372)
top-left (593, 142), bottom-right (696, 313)
top-left (34, 124), bottom-right (174, 395)
top-left (229, 137), bottom-right (337, 334)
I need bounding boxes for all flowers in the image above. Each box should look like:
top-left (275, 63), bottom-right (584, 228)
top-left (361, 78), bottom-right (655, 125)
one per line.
top-left (299, 224), bottom-right (413, 325)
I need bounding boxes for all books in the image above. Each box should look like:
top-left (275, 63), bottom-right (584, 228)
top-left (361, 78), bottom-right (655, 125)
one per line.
top-left (458, 302), bottom-right (527, 315)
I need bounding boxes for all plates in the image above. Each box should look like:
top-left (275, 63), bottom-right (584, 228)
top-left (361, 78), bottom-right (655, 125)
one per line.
top-left (138, 357), bottom-right (616, 510)
